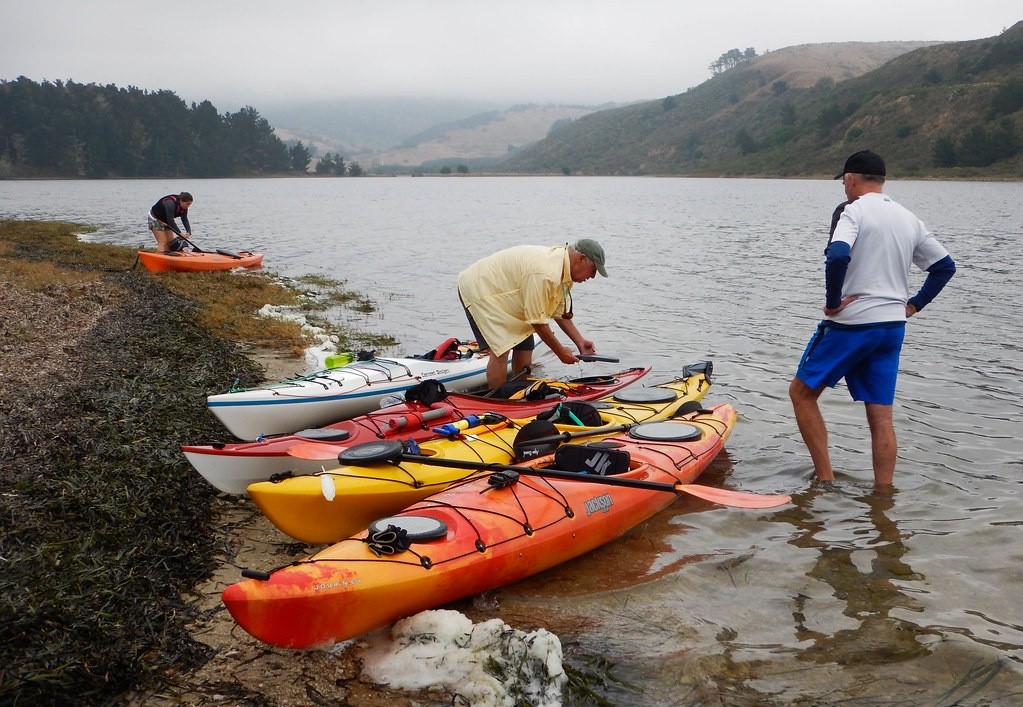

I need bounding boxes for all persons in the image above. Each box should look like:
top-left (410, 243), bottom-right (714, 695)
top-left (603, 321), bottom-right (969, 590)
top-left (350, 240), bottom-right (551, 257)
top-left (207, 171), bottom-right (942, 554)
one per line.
top-left (789, 148), bottom-right (956, 485)
top-left (457, 239), bottom-right (608, 390)
top-left (147, 192), bottom-right (194, 251)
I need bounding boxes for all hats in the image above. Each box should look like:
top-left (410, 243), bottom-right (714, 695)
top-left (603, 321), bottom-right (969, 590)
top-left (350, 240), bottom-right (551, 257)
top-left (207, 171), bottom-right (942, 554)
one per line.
top-left (575, 239), bottom-right (609, 278)
top-left (834, 149), bottom-right (886, 180)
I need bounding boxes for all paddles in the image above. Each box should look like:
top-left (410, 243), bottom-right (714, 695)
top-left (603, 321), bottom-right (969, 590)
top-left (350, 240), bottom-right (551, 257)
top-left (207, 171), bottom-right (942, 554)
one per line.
top-left (400, 453), bottom-right (792, 509)
top-left (514, 399), bottom-right (704, 462)
top-left (159, 221), bottom-right (203, 253)
top-left (483, 347), bottom-right (558, 399)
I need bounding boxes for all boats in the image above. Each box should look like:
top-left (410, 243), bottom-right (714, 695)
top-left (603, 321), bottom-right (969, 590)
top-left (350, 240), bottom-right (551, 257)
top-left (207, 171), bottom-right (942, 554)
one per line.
top-left (207, 333), bottom-right (549, 442)
top-left (179, 363), bottom-right (663, 496)
top-left (248, 361), bottom-right (715, 544)
top-left (137, 248), bottom-right (264, 273)
top-left (220, 402), bottom-right (737, 650)
